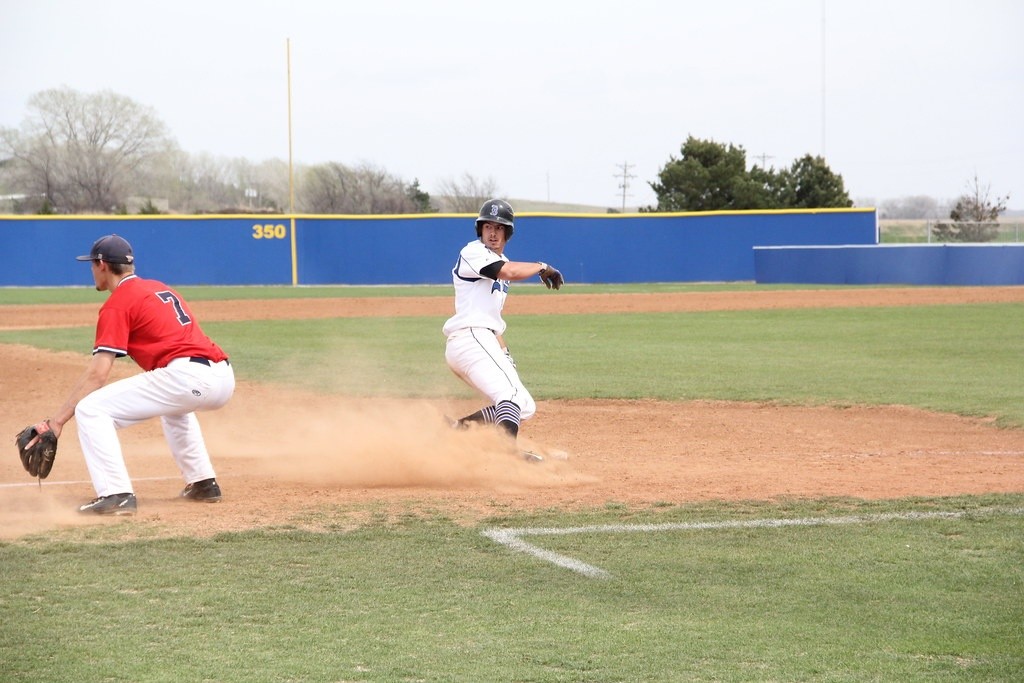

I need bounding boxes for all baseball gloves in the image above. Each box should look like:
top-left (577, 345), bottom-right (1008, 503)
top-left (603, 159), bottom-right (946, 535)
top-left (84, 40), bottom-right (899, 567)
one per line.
top-left (16, 420), bottom-right (58, 480)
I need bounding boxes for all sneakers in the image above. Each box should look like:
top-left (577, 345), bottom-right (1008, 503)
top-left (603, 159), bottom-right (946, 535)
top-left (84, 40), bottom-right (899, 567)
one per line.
top-left (445, 416), bottom-right (470, 431)
top-left (517, 448), bottom-right (545, 464)
top-left (79, 492), bottom-right (138, 517)
top-left (175, 478), bottom-right (223, 503)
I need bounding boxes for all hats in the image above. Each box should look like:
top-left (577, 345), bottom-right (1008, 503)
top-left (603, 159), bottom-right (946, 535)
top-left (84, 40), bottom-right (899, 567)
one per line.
top-left (76, 234), bottom-right (134, 265)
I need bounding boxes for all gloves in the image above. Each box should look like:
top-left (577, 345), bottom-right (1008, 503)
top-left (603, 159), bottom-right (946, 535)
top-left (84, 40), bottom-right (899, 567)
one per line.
top-left (502, 348), bottom-right (517, 369)
top-left (538, 261), bottom-right (565, 291)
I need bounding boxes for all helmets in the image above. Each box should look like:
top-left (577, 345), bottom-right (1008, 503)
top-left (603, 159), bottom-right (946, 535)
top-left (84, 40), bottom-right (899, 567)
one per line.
top-left (474, 198), bottom-right (514, 237)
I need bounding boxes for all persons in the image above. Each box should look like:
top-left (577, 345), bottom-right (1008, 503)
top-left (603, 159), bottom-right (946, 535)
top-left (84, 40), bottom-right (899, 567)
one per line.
top-left (15, 233), bottom-right (236, 517)
top-left (443, 199), bottom-right (565, 464)
